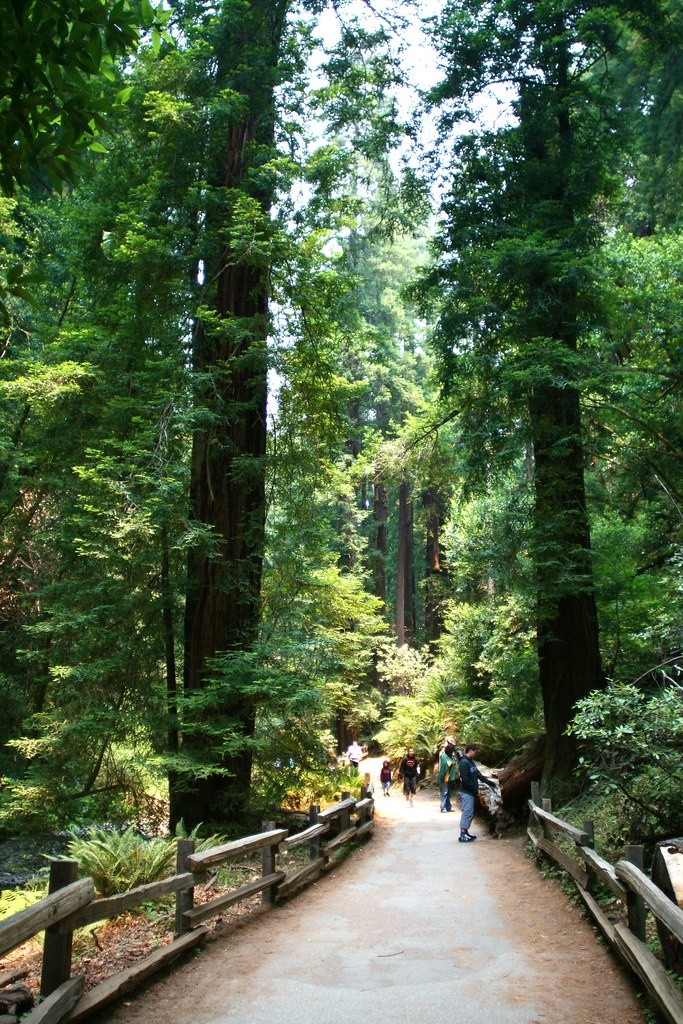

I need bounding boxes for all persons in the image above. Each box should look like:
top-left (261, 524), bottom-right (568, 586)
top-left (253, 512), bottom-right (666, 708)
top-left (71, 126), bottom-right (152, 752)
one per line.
top-left (347, 741), bottom-right (362, 778)
top-left (438, 740), bottom-right (456, 813)
top-left (398, 747), bottom-right (418, 808)
top-left (458, 744), bottom-right (495, 842)
top-left (379, 760), bottom-right (391, 797)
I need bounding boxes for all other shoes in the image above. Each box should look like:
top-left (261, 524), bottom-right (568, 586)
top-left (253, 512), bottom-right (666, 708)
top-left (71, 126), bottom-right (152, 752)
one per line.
top-left (405, 799), bottom-right (413, 808)
top-left (441, 807), bottom-right (455, 813)
top-left (383, 793), bottom-right (390, 797)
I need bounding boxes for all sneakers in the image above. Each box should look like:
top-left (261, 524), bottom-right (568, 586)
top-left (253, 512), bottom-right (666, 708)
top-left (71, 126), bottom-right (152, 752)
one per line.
top-left (458, 831), bottom-right (476, 842)
